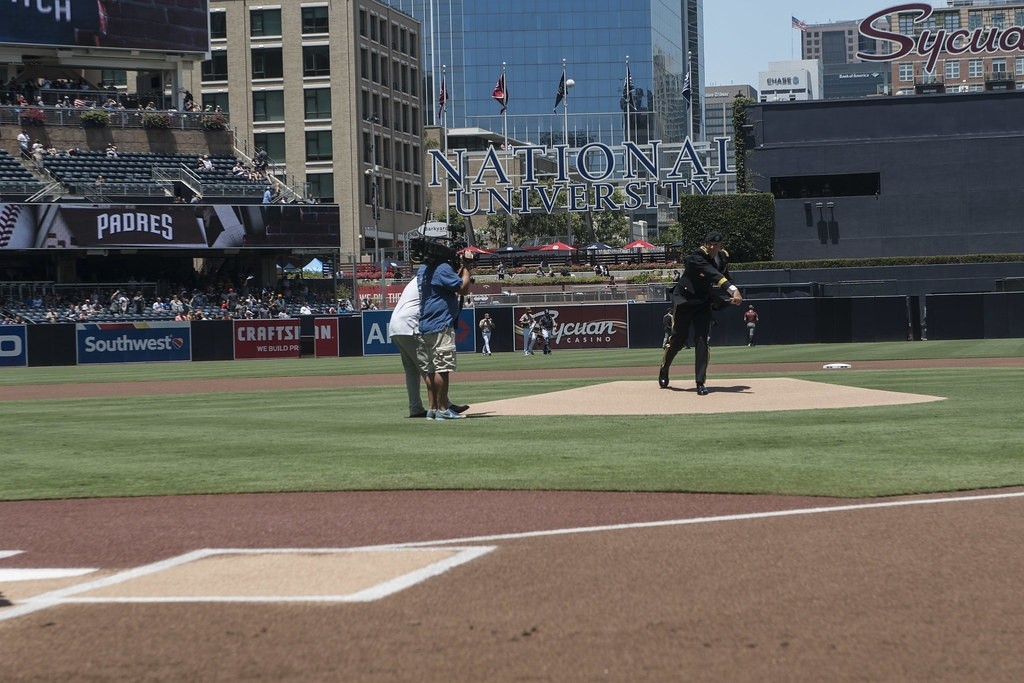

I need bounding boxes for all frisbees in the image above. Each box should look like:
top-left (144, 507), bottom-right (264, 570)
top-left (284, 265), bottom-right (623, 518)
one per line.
top-left (822, 363), bottom-right (852, 369)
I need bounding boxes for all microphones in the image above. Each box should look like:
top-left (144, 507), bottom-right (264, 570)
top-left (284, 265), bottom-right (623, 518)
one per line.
top-left (454, 241), bottom-right (468, 247)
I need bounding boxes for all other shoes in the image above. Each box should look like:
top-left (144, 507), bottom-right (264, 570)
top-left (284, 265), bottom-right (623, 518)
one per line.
top-left (450, 405), bottom-right (469, 413)
top-left (524, 351), bottom-right (529, 355)
top-left (530, 351), bottom-right (534, 355)
top-left (412, 411), bottom-right (427, 418)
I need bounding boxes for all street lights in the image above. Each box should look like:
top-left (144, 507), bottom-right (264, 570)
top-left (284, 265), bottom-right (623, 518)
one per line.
top-left (365, 113), bottom-right (380, 262)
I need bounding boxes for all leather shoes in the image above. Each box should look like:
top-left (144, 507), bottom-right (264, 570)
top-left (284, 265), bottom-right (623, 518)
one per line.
top-left (696, 384), bottom-right (708, 395)
top-left (659, 370), bottom-right (669, 387)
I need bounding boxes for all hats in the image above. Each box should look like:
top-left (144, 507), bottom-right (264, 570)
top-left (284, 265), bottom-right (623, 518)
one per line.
top-left (525, 307), bottom-right (532, 312)
top-left (705, 232), bottom-right (721, 243)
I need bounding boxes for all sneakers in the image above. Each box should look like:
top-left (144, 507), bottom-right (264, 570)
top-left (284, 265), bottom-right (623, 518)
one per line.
top-left (426, 409), bottom-right (437, 420)
top-left (435, 408), bottom-right (466, 420)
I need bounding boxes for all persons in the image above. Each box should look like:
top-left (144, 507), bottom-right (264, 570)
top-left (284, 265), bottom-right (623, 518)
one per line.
top-left (594, 263), bottom-right (609, 276)
top-left (658, 231), bottom-right (742, 396)
top-left (673, 269), bottom-right (680, 282)
top-left (497, 261), bottom-right (504, 280)
top-left (519, 307), bottom-right (554, 356)
top-left (662, 307), bottom-right (673, 348)
top-left (9, 75), bottom-right (318, 204)
top-left (3, 274), bottom-right (379, 325)
top-left (609, 276), bottom-right (615, 285)
top-left (744, 305), bottom-right (759, 346)
top-left (479, 314), bottom-right (495, 356)
top-left (508, 271), bottom-right (516, 279)
top-left (388, 239), bottom-right (470, 421)
top-left (537, 266), bottom-right (570, 278)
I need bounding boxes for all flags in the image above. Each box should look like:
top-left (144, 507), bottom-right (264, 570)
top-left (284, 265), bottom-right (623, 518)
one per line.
top-left (553, 72), bottom-right (568, 114)
top-left (681, 64), bottom-right (690, 110)
top-left (492, 74), bottom-right (509, 115)
top-left (622, 68), bottom-right (637, 111)
top-left (438, 78), bottom-right (449, 119)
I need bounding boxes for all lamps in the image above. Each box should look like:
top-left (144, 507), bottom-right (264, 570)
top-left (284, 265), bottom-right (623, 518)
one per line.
top-left (742, 125), bottom-right (754, 133)
top-left (816, 201), bottom-right (824, 208)
top-left (827, 201), bottom-right (835, 208)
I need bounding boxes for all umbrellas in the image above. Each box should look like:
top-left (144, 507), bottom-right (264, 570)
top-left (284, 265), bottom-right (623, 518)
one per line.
top-left (457, 240), bottom-right (656, 267)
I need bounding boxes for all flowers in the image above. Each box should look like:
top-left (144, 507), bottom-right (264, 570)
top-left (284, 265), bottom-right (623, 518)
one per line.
top-left (202, 114), bottom-right (230, 130)
top-left (19, 108), bottom-right (46, 127)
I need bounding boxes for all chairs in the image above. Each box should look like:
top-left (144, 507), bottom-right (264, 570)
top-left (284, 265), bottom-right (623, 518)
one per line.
top-left (0, 281), bottom-right (356, 328)
top-left (0, 148), bottom-right (321, 204)
